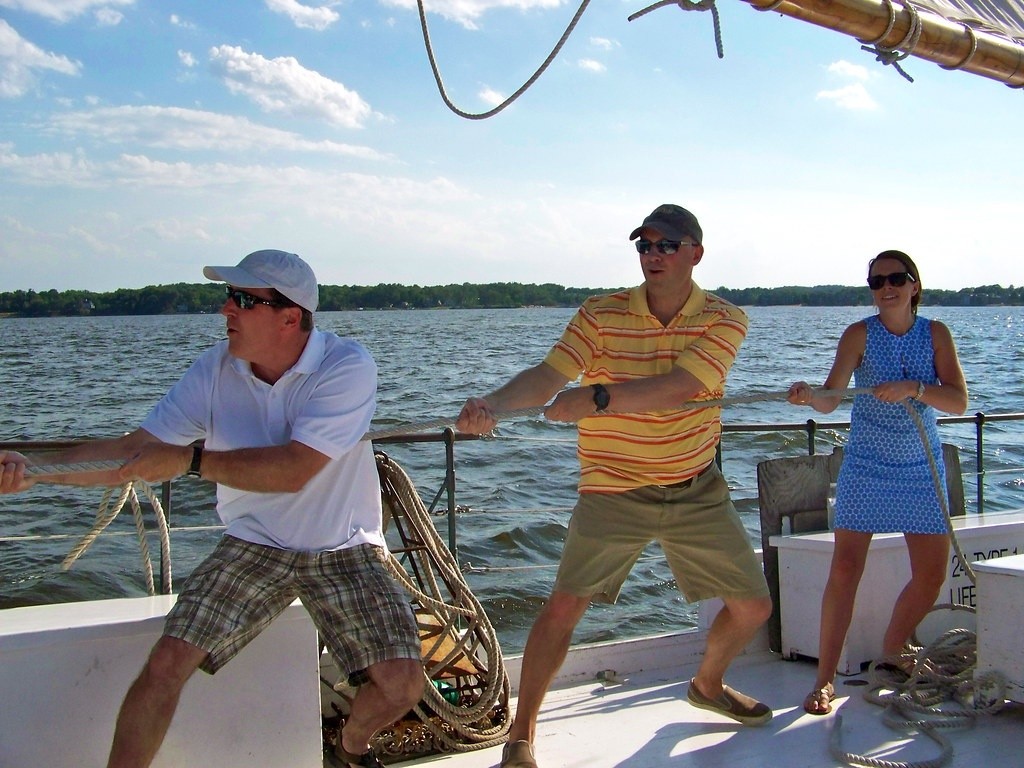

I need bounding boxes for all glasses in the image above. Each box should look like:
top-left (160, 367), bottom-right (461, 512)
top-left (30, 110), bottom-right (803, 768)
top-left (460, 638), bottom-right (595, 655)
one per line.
top-left (867, 271), bottom-right (915, 290)
top-left (225, 284), bottom-right (293, 310)
top-left (635, 238), bottom-right (699, 255)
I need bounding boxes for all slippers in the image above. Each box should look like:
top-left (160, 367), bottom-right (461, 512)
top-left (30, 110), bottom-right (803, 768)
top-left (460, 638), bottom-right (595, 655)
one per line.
top-left (883, 660), bottom-right (930, 683)
top-left (805, 688), bottom-right (840, 714)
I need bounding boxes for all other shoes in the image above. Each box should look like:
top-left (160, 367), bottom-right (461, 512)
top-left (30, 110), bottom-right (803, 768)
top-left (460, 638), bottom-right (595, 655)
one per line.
top-left (687, 676), bottom-right (773, 727)
top-left (501, 740), bottom-right (539, 767)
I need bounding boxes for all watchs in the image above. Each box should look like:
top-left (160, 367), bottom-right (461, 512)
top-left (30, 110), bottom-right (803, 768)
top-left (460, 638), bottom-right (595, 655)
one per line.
top-left (186, 447), bottom-right (202, 480)
top-left (913, 380), bottom-right (925, 401)
top-left (589, 383), bottom-right (611, 411)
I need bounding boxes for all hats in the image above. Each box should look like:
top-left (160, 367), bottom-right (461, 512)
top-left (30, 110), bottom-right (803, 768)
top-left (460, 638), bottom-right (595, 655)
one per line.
top-left (629, 204), bottom-right (704, 246)
top-left (203, 250), bottom-right (319, 314)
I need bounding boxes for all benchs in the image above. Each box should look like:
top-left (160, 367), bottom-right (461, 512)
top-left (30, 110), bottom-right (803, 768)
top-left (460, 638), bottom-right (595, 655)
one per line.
top-left (0, 594), bottom-right (324, 768)
top-left (971, 553), bottom-right (1024, 712)
top-left (769, 510), bottom-right (1024, 676)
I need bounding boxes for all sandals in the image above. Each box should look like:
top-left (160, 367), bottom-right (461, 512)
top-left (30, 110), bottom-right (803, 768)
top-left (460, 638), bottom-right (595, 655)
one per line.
top-left (324, 718), bottom-right (387, 768)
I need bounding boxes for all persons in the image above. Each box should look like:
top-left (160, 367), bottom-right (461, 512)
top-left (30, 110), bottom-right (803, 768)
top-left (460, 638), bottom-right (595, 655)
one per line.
top-left (0, 249), bottom-right (425, 768)
top-left (456, 204), bottom-right (773, 768)
top-left (785, 249), bottom-right (969, 716)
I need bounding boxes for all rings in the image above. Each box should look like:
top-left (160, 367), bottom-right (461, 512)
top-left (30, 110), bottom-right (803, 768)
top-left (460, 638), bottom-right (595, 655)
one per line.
top-left (800, 400), bottom-right (805, 403)
